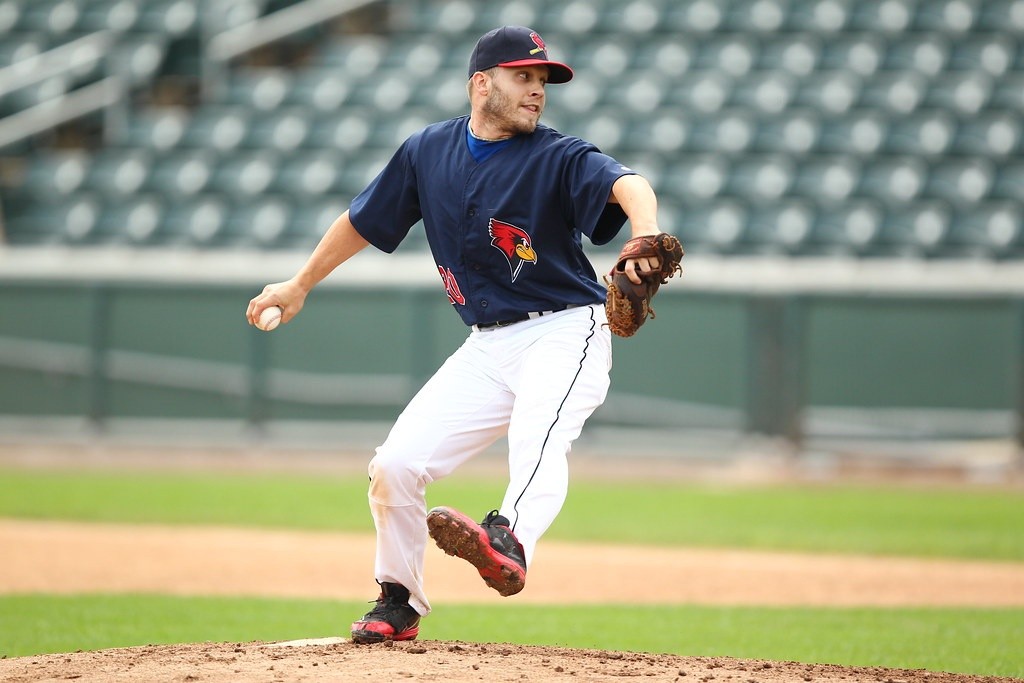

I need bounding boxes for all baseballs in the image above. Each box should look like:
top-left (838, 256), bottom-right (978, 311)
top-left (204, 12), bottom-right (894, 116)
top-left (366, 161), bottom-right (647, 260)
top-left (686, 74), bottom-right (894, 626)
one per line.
top-left (251, 306), bottom-right (283, 332)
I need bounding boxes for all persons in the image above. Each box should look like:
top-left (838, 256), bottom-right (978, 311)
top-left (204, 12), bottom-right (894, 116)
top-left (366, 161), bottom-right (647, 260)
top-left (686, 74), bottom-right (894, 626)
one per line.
top-left (246, 25), bottom-right (683, 643)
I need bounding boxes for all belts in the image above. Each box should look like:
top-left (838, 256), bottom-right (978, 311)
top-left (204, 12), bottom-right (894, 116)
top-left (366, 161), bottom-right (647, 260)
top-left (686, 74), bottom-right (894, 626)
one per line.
top-left (478, 305), bottom-right (567, 328)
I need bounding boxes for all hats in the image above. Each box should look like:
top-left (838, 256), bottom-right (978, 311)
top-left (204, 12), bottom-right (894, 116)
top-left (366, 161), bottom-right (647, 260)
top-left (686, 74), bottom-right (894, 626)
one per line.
top-left (468, 26), bottom-right (574, 84)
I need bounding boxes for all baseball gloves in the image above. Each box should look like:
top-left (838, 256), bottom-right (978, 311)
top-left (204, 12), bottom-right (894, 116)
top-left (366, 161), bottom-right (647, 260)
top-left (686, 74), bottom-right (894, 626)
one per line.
top-left (598, 232), bottom-right (686, 338)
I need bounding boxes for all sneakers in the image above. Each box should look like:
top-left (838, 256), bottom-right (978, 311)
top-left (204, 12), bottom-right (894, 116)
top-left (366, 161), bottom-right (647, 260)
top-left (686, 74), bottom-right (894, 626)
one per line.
top-left (350, 578), bottom-right (420, 643)
top-left (426, 505), bottom-right (527, 598)
